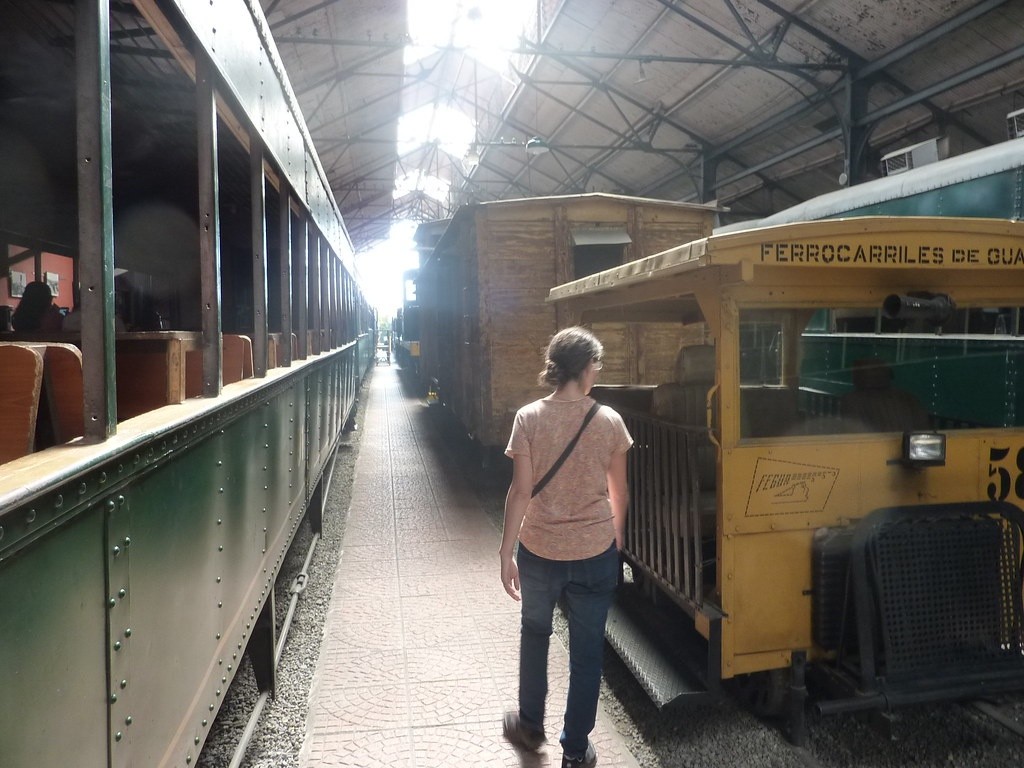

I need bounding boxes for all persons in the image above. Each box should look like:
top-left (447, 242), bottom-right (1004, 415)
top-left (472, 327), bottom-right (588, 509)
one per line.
top-left (499, 327), bottom-right (634, 768)
top-left (62, 291), bottom-right (125, 333)
top-left (12, 281), bottom-right (62, 331)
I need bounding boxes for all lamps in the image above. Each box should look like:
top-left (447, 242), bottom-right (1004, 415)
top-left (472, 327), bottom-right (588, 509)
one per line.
top-left (524, 136), bottom-right (550, 157)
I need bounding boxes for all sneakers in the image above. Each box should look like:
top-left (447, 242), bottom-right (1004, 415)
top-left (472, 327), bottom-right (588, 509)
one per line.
top-left (561, 740), bottom-right (597, 768)
top-left (504, 712), bottom-right (546, 749)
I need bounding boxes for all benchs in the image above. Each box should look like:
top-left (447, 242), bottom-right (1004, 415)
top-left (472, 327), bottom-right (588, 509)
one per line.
top-left (593, 384), bottom-right (804, 491)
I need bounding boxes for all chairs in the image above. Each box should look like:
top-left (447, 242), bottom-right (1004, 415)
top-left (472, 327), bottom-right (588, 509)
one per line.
top-left (652, 344), bottom-right (757, 560)
top-left (845, 359), bottom-right (928, 438)
top-left (0, 328), bottom-right (332, 466)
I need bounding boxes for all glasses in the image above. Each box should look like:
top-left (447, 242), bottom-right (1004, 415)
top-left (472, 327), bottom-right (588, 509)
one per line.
top-left (591, 356), bottom-right (604, 370)
